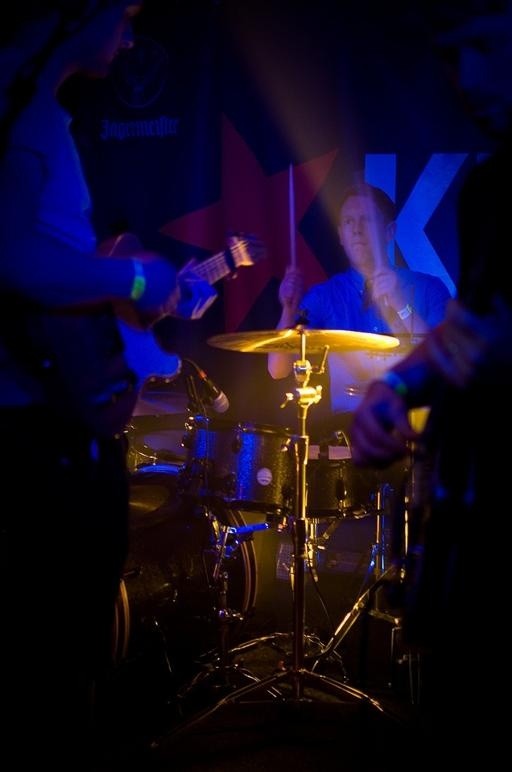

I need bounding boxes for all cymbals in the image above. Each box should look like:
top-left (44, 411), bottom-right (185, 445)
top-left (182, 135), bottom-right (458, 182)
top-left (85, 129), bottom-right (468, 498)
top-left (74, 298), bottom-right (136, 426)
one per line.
top-left (204, 328), bottom-right (412, 358)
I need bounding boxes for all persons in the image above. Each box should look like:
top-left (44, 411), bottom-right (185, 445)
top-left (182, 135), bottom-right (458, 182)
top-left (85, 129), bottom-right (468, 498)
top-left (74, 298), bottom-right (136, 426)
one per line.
top-left (2, 0), bottom-right (226, 770)
top-left (333, 1), bottom-right (509, 772)
top-left (274, 182), bottom-right (456, 341)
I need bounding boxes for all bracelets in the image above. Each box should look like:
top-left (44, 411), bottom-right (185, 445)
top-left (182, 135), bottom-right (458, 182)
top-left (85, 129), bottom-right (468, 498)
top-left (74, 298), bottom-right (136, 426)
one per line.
top-left (378, 365), bottom-right (414, 411)
top-left (396, 303), bottom-right (417, 320)
top-left (126, 257), bottom-right (146, 302)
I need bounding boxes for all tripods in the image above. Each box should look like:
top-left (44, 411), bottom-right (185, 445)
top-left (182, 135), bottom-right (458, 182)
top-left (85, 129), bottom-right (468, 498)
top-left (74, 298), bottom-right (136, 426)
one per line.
top-left (156, 326), bottom-right (422, 753)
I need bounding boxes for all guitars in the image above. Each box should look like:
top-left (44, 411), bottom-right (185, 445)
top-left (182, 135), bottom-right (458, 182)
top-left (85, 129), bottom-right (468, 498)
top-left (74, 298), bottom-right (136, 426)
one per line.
top-left (34, 231), bottom-right (268, 438)
top-left (406, 229), bottom-right (507, 594)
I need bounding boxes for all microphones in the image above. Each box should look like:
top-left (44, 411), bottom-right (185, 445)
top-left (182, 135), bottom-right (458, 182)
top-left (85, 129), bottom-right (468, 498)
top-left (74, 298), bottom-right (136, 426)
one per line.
top-left (169, 354), bottom-right (232, 443)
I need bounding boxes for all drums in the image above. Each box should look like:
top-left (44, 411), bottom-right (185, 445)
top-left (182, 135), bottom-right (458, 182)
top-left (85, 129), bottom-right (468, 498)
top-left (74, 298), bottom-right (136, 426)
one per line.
top-left (180, 412), bottom-right (294, 508)
top-left (120, 414), bottom-right (188, 483)
top-left (296, 449), bottom-right (372, 518)
top-left (182, 631), bottom-right (348, 719)
top-left (126, 495), bottom-right (298, 663)
